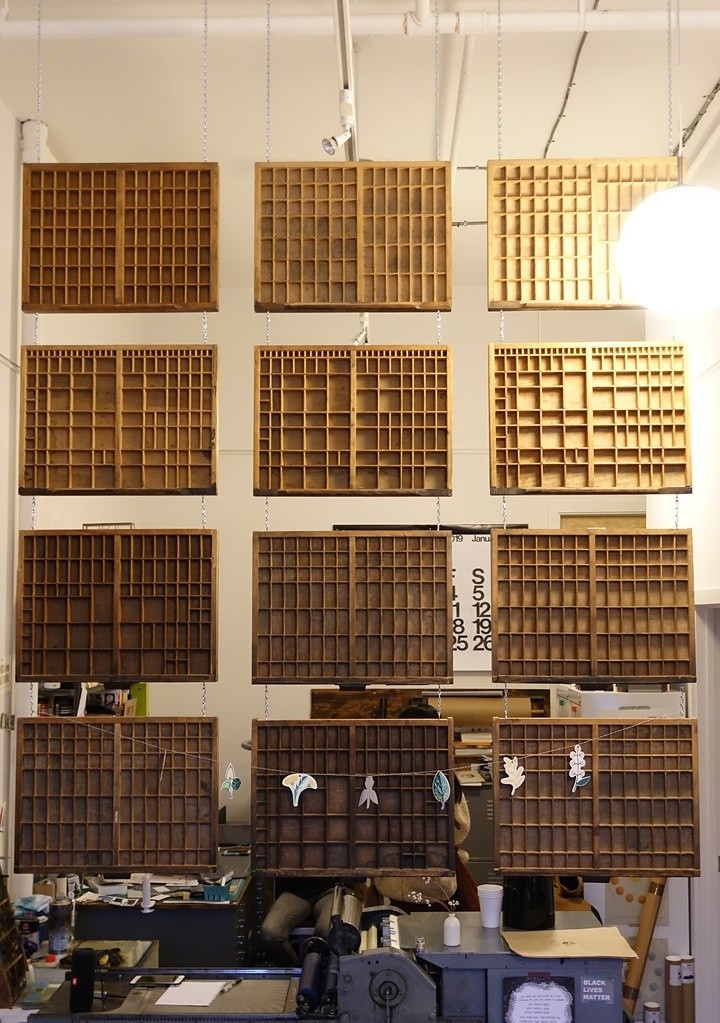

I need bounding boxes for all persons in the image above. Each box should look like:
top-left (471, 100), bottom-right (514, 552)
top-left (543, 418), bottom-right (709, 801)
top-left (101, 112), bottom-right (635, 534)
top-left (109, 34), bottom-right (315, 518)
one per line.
top-left (258, 871), bottom-right (366, 965)
top-left (366, 705), bottom-right (486, 910)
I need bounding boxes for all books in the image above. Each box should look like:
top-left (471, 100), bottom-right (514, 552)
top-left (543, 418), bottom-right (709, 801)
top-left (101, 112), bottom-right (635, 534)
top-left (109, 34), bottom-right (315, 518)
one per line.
top-left (454, 769), bottom-right (485, 789)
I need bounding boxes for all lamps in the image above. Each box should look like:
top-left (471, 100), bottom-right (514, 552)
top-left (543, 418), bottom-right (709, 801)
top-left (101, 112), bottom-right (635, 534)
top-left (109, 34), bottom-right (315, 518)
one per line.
top-left (318, 122), bottom-right (355, 156)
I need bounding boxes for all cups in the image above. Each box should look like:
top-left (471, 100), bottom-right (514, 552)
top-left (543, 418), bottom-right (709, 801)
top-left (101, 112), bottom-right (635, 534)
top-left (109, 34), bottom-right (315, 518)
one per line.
top-left (477, 883), bottom-right (503, 929)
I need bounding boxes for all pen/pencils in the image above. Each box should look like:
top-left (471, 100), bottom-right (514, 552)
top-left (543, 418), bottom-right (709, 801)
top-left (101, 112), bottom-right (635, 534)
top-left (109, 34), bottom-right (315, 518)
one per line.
top-left (219, 976), bottom-right (245, 993)
